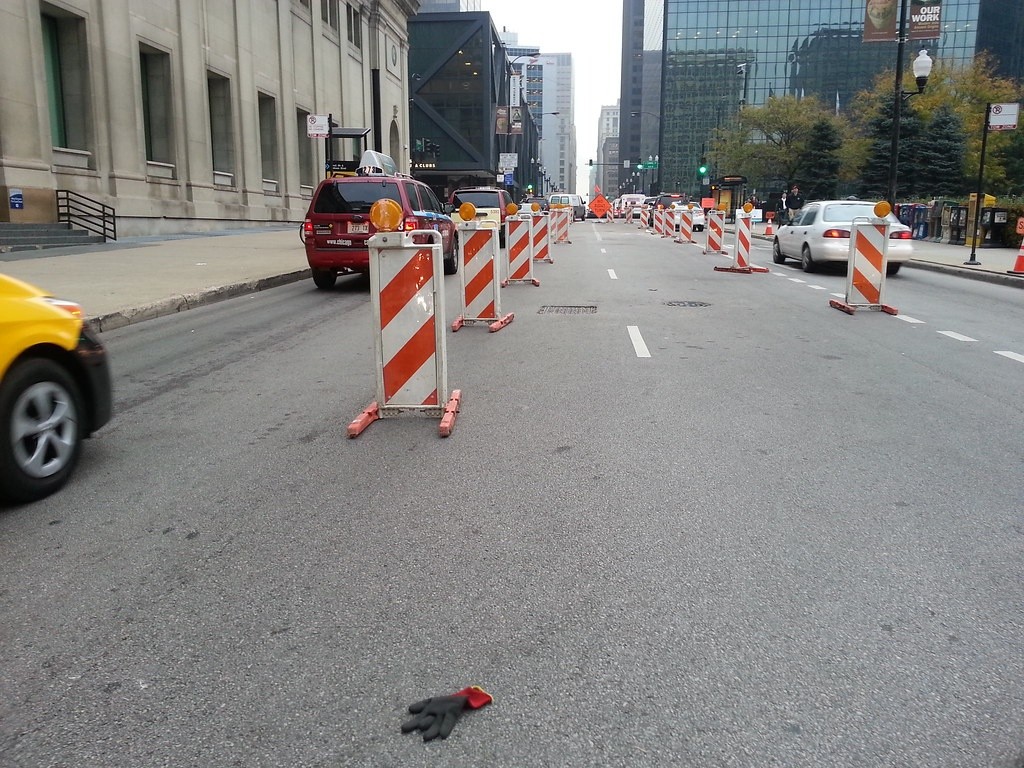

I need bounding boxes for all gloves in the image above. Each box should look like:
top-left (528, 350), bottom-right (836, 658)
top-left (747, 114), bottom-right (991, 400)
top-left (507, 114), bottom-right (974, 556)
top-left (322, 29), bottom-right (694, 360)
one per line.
top-left (401, 685), bottom-right (493, 740)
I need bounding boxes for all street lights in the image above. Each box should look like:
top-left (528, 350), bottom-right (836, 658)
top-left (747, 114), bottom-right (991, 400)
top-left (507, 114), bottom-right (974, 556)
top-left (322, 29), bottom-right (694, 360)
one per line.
top-left (554, 187), bottom-right (559, 192)
top-left (530, 111), bottom-right (560, 159)
top-left (735, 62), bottom-right (748, 150)
top-left (507, 53), bottom-right (541, 153)
top-left (549, 181), bottom-right (555, 193)
top-left (628, 110), bottom-right (662, 193)
top-left (531, 158), bottom-right (540, 197)
top-left (648, 154), bottom-right (659, 196)
top-left (544, 174), bottom-right (551, 193)
top-left (410, 73), bottom-right (421, 175)
top-left (552, 184), bottom-right (557, 192)
top-left (539, 166), bottom-right (545, 197)
top-left (890, 42), bottom-right (933, 216)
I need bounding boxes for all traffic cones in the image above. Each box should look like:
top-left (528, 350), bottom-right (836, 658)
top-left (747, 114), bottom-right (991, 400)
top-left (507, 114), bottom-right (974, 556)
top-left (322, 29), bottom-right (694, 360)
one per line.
top-left (761, 217), bottom-right (774, 236)
top-left (1007, 237), bottom-right (1024, 274)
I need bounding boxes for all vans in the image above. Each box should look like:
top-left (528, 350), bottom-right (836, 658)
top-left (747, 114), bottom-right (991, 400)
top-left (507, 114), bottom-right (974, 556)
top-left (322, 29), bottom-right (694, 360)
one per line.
top-left (617, 193), bottom-right (647, 219)
top-left (547, 194), bottom-right (586, 222)
top-left (611, 199), bottom-right (620, 218)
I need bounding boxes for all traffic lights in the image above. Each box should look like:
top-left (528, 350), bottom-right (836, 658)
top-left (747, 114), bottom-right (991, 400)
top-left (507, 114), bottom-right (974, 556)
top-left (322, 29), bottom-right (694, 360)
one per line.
top-left (422, 137), bottom-right (430, 153)
top-left (432, 143), bottom-right (440, 158)
top-left (638, 158), bottom-right (643, 171)
top-left (697, 157), bottom-right (708, 175)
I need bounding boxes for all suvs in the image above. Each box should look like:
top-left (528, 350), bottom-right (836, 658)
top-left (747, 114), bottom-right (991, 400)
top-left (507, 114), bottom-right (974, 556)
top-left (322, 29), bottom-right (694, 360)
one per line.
top-left (643, 197), bottom-right (656, 225)
top-left (299, 148), bottom-right (459, 291)
top-left (446, 186), bottom-right (517, 247)
top-left (527, 198), bottom-right (549, 215)
top-left (650, 193), bottom-right (689, 227)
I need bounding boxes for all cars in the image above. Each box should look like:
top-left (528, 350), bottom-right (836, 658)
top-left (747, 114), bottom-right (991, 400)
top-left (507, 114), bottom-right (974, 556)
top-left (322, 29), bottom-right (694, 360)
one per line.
top-left (0, 269), bottom-right (111, 502)
top-left (669, 202), bottom-right (703, 232)
top-left (771, 199), bottom-right (912, 274)
top-left (517, 203), bottom-right (548, 215)
